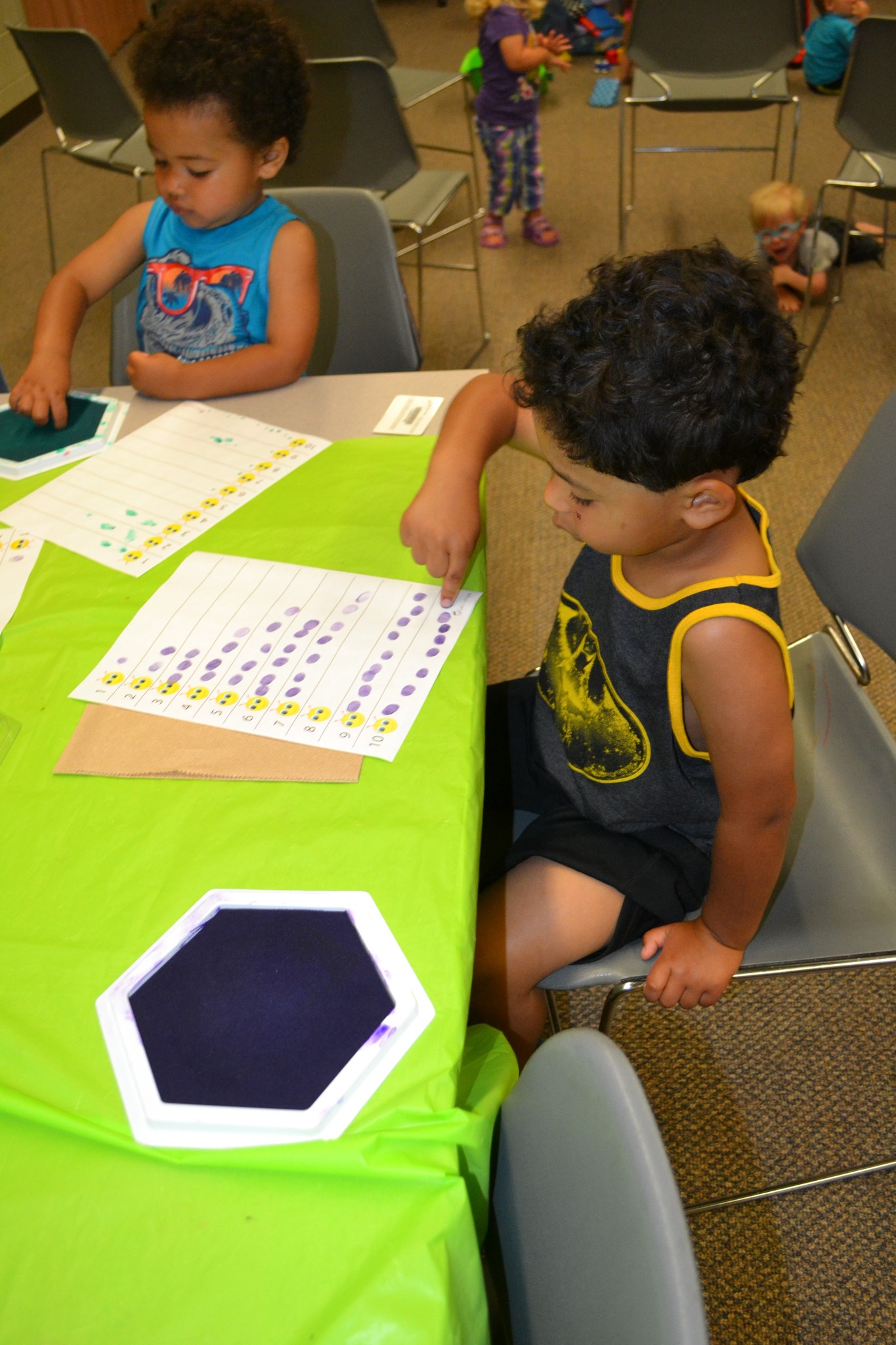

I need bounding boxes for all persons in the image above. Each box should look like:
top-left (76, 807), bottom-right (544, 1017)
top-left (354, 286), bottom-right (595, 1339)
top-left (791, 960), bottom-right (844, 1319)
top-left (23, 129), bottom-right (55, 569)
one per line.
top-left (7, 0), bottom-right (323, 434)
top-left (402, 238), bottom-right (804, 1062)
top-left (746, 181), bottom-right (888, 307)
top-left (800, 0), bottom-right (870, 94)
top-left (464, 1), bottom-right (574, 246)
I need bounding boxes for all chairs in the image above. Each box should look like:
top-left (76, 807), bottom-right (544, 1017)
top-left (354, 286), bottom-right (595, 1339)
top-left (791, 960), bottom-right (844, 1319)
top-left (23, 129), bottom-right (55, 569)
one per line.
top-left (259, 53), bottom-right (494, 375)
top-left (254, 0), bottom-right (488, 260)
top-left (3, 20), bottom-right (160, 280)
top-left (253, 184), bottom-right (425, 379)
top-left (489, 1022), bottom-right (716, 1344)
top-left (616, 0), bottom-right (804, 239)
top-left (530, 389), bottom-right (895, 1218)
top-left (792, 17), bottom-right (896, 305)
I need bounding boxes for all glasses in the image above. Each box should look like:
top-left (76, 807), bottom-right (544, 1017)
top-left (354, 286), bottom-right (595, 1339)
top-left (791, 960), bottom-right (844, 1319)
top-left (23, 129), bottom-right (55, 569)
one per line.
top-left (756, 222), bottom-right (802, 243)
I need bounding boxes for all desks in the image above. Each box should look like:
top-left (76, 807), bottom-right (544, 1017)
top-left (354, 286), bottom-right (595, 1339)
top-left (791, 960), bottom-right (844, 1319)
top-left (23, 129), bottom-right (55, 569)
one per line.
top-left (0, 363), bottom-right (493, 1345)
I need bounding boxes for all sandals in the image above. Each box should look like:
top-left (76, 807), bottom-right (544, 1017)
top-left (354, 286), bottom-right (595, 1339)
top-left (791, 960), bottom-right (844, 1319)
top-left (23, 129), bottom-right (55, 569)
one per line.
top-left (479, 218), bottom-right (506, 248)
top-left (524, 216), bottom-right (560, 246)
